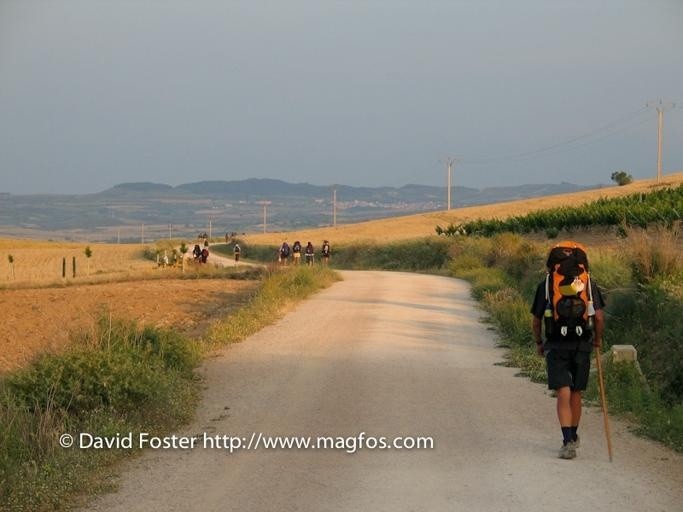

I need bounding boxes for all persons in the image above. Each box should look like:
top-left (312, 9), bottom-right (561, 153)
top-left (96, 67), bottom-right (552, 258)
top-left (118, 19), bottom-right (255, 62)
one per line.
top-left (293, 241), bottom-right (301, 264)
top-left (321, 240), bottom-right (330, 267)
top-left (192, 232), bottom-right (210, 264)
top-left (233, 244), bottom-right (241, 261)
top-left (279, 242), bottom-right (289, 265)
top-left (530, 240), bottom-right (606, 459)
top-left (306, 242), bottom-right (313, 266)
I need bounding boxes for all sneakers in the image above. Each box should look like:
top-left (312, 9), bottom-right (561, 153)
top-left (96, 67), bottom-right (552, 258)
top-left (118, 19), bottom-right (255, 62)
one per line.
top-left (558, 434), bottom-right (581, 459)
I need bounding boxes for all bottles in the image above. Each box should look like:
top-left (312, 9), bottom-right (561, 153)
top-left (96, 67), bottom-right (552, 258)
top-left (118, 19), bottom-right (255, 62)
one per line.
top-left (544, 310), bottom-right (555, 339)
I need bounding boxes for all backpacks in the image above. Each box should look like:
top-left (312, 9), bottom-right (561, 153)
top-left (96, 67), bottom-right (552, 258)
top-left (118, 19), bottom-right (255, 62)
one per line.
top-left (282, 243), bottom-right (330, 257)
top-left (235, 246), bottom-right (240, 252)
top-left (543, 240), bottom-right (597, 342)
top-left (194, 245), bottom-right (209, 257)
